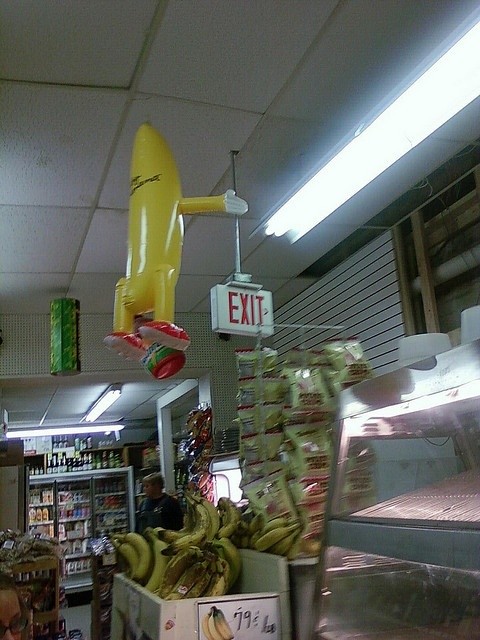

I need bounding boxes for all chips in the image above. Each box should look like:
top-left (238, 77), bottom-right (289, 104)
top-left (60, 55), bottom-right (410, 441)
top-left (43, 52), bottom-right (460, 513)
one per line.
top-left (234, 339), bottom-right (373, 541)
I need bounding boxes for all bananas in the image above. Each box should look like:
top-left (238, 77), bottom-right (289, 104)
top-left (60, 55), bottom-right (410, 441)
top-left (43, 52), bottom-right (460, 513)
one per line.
top-left (113, 491), bottom-right (305, 600)
top-left (202, 606), bottom-right (235, 639)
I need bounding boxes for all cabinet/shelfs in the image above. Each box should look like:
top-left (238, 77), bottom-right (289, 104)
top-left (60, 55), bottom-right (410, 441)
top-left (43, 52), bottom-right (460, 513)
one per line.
top-left (0, 534), bottom-right (60, 640)
top-left (311, 338), bottom-right (480, 640)
top-left (56, 470), bottom-right (93, 593)
top-left (93, 464), bottom-right (135, 541)
top-left (89, 546), bottom-right (137, 640)
top-left (25, 466), bottom-right (56, 539)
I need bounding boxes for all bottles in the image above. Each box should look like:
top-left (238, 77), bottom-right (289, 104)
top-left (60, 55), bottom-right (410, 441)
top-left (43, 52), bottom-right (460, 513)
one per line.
top-left (63, 435), bottom-right (70, 448)
top-left (57, 489), bottom-right (93, 574)
top-left (93, 478), bottom-right (130, 536)
top-left (73, 434), bottom-right (94, 451)
top-left (58, 436), bottom-right (64, 448)
top-left (29, 485), bottom-right (54, 539)
top-left (52, 435), bottom-right (58, 448)
top-left (29, 451), bottom-right (125, 475)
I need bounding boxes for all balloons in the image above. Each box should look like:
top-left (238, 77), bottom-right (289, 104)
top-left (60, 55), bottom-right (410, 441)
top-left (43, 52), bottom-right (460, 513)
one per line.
top-left (104, 125), bottom-right (248, 361)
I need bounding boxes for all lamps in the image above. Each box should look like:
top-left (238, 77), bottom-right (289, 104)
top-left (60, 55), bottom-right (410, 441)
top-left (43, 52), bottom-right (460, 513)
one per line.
top-left (250, 21), bottom-right (480, 249)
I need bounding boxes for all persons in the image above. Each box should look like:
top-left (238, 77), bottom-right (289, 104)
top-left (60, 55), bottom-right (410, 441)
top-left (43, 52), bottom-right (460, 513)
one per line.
top-left (1, 584), bottom-right (28, 639)
top-left (135, 474), bottom-right (182, 531)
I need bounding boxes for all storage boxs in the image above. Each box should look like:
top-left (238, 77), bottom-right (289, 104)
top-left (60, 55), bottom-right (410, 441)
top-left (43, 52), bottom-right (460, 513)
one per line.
top-left (108, 569), bottom-right (281, 640)
